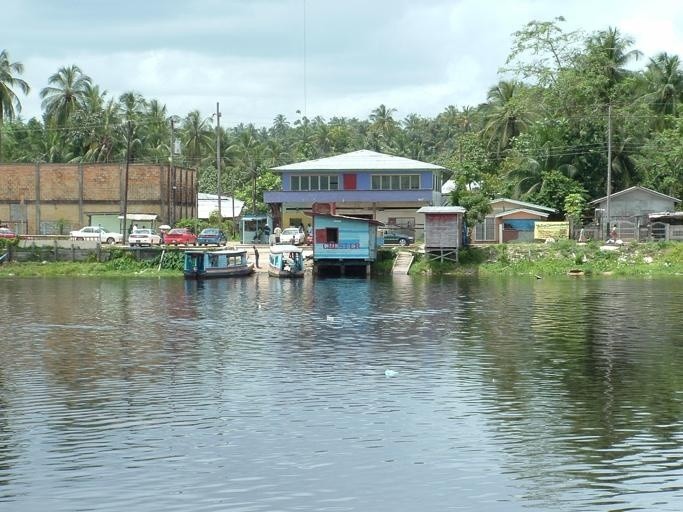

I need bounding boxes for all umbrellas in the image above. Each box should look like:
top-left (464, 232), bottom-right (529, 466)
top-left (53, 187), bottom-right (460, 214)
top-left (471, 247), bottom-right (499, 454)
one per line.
top-left (158, 224), bottom-right (172, 230)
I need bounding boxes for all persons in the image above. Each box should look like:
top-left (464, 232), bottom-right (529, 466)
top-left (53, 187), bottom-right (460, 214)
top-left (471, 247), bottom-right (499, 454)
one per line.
top-left (290, 236), bottom-right (295, 245)
top-left (579, 226), bottom-right (587, 243)
top-left (305, 223), bottom-right (314, 246)
top-left (127, 223), bottom-right (145, 233)
top-left (289, 223), bottom-right (295, 228)
top-left (160, 229), bottom-right (165, 238)
top-left (264, 224), bottom-right (271, 244)
top-left (253, 246), bottom-right (259, 268)
top-left (274, 223), bottom-right (282, 244)
top-left (610, 224), bottom-right (617, 241)
top-left (251, 227), bottom-right (262, 244)
top-left (298, 223), bottom-right (303, 233)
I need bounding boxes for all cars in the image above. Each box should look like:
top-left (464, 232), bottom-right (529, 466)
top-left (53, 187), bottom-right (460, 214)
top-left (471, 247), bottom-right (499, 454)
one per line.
top-left (70, 225), bottom-right (122, 245)
top-left (280, 228), bottom-right (313, 245)
top-left (384, 233), bottom-right (413, 247)
top-left (164, 228), bottom-right (196, 246)
top-left (0, 227), bottom-right (15, 238)
top-left (198, 228), bottom-right (227, 247)
top-left (128, 229), bottom-right (162, 246)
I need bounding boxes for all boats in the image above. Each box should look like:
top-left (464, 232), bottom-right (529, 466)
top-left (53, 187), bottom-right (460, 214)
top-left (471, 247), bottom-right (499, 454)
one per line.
top-left (268, 245), bottom-right (303, 278)
top-left (183, 250), bottom-right (254, 277)
